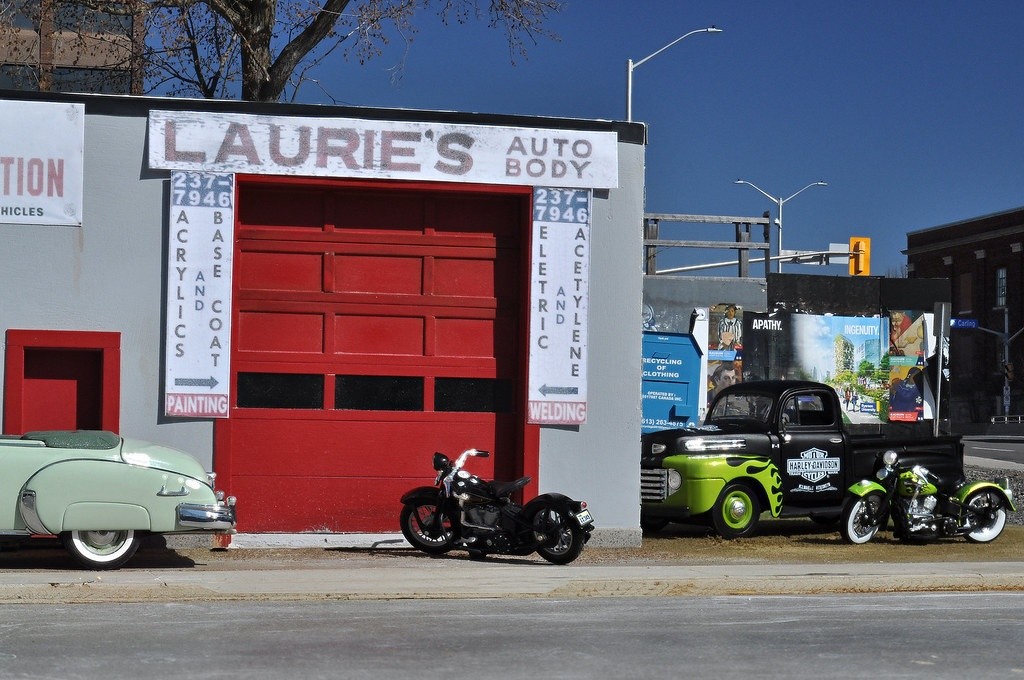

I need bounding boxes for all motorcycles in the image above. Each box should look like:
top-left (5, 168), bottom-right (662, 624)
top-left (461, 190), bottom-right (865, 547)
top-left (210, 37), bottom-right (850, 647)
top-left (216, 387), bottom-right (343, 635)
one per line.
top-left (841, 448), bottom-right (1017, 546)
top-left (397, 448), bottom-right (596, 567)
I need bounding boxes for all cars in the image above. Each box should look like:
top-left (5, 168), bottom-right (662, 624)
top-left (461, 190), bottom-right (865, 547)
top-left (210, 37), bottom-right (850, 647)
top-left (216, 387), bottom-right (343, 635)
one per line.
top-left (0, 431), bottom-right (241, 567)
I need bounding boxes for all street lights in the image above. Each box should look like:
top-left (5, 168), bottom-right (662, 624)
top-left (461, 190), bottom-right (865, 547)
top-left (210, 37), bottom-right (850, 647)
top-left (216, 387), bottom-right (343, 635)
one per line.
top-left (624, 25), bottom-right (724, 127)
top-left (733, 179), bottom-right (829, 273)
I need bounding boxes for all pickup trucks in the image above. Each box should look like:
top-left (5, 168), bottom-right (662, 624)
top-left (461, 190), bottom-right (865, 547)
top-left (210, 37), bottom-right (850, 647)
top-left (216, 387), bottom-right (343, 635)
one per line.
top-left (640, 379), bottom-right (965, 541)
